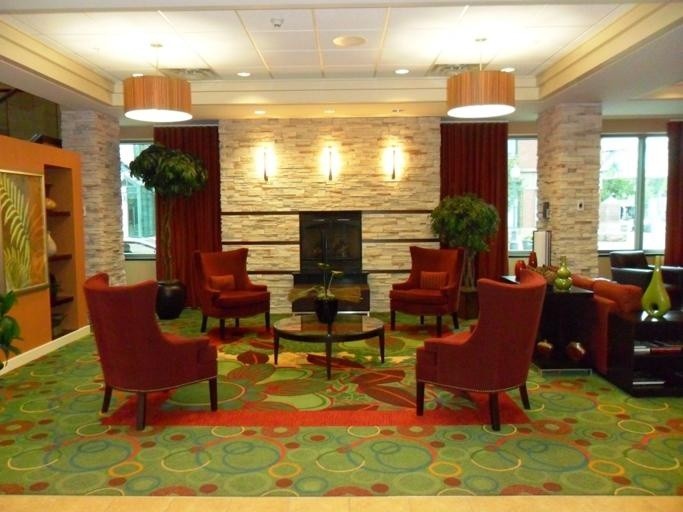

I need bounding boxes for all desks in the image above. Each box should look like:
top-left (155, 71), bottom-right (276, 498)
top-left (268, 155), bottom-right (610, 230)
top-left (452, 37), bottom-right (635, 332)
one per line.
top-left (502, 274), bottom-right (595, 377)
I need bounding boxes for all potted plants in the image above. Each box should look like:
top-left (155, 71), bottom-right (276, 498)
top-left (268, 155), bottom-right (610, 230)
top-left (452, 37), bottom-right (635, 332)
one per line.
top-left (288, 262), bottom-right (345, 324)
top-left (420, 193), bottom-right (502, 321)
top-left (129, 144), bottom-right (208, 319)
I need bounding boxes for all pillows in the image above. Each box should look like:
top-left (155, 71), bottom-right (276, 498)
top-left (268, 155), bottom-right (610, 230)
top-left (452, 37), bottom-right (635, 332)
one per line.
top-left (209, 274), bottom-right (234, 291)
top-left (419, 272), bottom-right (446, 288)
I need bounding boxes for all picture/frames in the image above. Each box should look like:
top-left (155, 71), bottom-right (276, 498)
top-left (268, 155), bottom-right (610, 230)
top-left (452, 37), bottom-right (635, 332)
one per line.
top-left (0, 169), bottom-right (51, 296)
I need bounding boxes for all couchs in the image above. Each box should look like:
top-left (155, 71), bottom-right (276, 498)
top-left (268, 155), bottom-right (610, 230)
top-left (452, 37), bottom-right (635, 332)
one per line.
top-left (570, 271), bottom-right (683, 398)
top-left (608, 251), bottom-right (683, 302)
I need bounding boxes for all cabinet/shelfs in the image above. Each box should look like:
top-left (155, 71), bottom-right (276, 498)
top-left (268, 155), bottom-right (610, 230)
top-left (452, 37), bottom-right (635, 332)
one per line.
top-left (0, 135), bottom-right (90, 379)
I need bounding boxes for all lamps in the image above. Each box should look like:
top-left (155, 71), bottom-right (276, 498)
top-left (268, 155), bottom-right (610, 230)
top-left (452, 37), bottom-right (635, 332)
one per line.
top-left (447, 35), bottom-right (516, 120)
top-left (122, 42), bottom-right (192, 124)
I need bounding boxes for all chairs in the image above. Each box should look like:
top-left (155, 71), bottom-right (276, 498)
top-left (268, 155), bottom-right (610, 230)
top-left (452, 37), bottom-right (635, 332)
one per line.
top-left (389, 246), bottom-right (464, 336)
top-left (82, 273), bottom-right (220, 431)
top-left (192, 248), bottom-right (271, 339)
top-left (415, 268), bottom-right (547, 431)
